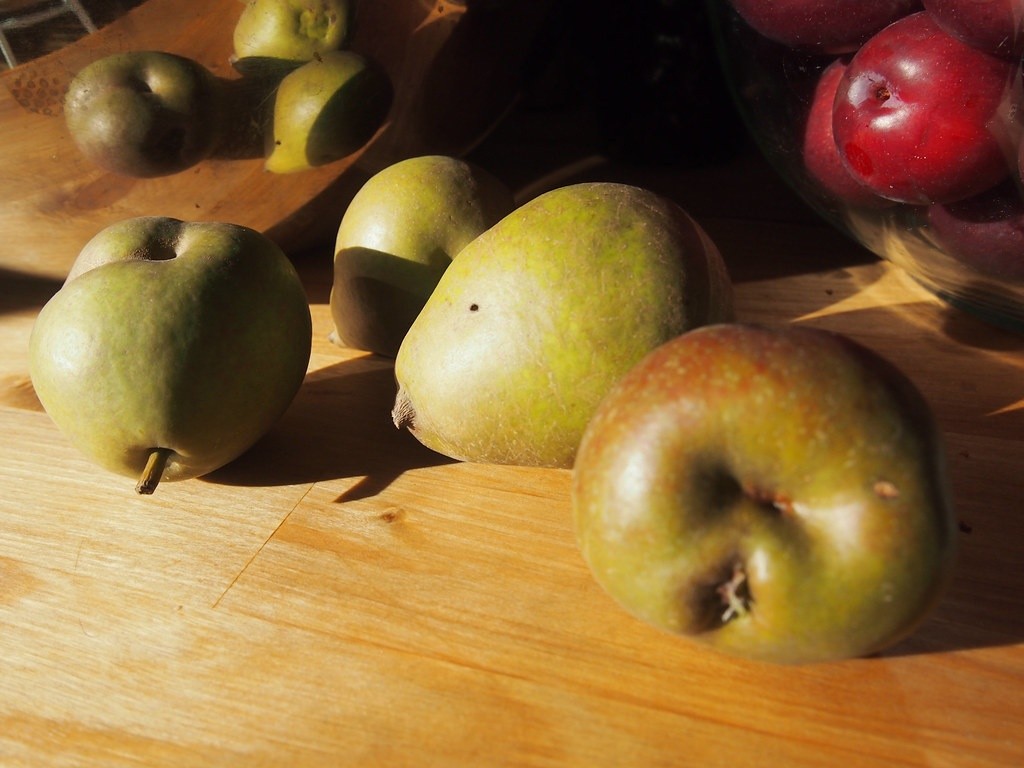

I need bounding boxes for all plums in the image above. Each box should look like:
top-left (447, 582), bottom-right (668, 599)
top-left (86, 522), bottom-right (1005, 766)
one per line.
top-left (725, 0), bottom-right (1024, 282)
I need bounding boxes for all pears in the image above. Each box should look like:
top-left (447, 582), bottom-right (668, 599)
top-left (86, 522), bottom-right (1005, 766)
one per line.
top-left (26, 0), bottom-right (945, 663)
